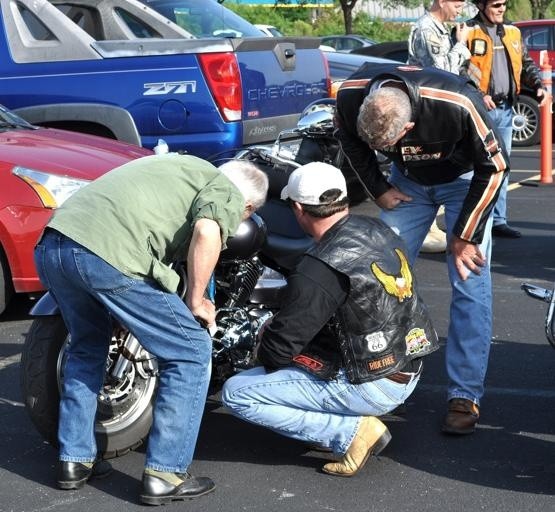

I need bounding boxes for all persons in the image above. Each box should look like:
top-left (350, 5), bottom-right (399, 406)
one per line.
top-left (336, 60), bottom-right (512, 436)
top-left (408, 0), bottom-right (472, 253)
top-left (221, 161), bottom-right (440, 478)
top-left (450, 0), bottom-right (549, 239)
top-left (32, 153), bottom-right (270, 504)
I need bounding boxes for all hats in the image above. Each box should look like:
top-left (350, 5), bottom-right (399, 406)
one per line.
top-left (280, 161), bottom-right (348, 206)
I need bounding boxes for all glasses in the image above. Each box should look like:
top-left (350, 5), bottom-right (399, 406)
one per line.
top-left (492, 2), bottom-right (506, 7)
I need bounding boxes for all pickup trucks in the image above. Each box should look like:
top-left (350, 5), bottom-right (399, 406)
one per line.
top-left (0, 0), bottom-right (333, 150)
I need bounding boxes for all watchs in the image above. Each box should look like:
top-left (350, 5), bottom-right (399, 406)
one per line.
top-left (456, 40), bottom-right (469, 48)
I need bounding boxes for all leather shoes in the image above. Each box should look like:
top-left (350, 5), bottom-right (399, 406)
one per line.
top-left (321, 416), bottom-right (392, 477)
top-left (440, 399), bottom-right (481, 435)
top-left (138, 468), bottom-right (216, 506)
top-left (56, 460), bottom-right (113, 490)
top-left (492, 224), bottom-right (520, 238)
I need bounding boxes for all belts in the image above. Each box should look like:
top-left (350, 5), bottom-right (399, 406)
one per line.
top-left (386, 372), bottom-right (411, 384)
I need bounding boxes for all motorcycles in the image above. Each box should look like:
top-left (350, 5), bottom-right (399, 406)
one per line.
top-left (18, 232), bottom-right (276, 458)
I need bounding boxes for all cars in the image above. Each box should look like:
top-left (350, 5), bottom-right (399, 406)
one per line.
top-left (0, 106), bottom-right (155, 315)
top-left (506, 15), bottom-right (555, 87)
top-left (319, 32), bottom-right (541, 145)
top-left (251, 24), bottom-right (282, 41)
